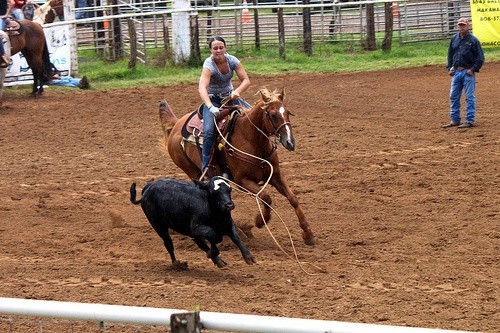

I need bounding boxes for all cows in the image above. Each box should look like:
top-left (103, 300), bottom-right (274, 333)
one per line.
top-left (129, 173), bottom-right (257, 268)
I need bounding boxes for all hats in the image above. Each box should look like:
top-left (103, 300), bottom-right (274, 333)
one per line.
top-left (458, 18), bottom-right (468, 25)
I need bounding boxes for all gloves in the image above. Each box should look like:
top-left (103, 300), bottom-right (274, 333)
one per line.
top-left (209, 105), bottom-right (220, 118)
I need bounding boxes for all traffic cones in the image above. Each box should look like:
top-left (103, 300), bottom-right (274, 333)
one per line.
top-left (102, 11), bottom-right (109, 29)
top-left (392, 1), bottom-right (400, 18)
top-left (241, 0), bottom-right (253, 24)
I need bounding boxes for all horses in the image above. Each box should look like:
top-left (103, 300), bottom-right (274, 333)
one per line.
top-left (4, 19), bottom-right (51, 96)
top-left (159, 85), bottom-right (317, 246)
top-left (33, 0), bottom-right (63, 26)
top-left (0, 29), bottom-right (12, 88)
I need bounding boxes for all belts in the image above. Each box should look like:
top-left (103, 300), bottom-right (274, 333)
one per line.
top-left (457, 66), bottom-right (464, 70)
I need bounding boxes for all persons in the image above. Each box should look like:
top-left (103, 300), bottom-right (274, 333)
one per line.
top-left (11, 0), bottom-right (28, 21)
top-left (442, 19), bottom-right (485, 127)
top-left (47, 0), bottom-right (64, 21)
top-left (0, 0), bottom-right (16, 68)
top-left (199, 37), bottom-right (252, 178)
top-left (75, 0), bottom-right (92, 28)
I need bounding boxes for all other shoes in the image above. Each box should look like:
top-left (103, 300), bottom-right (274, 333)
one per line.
top-left (442, 123), bottom-right (462, 128)
top-left (457, 122), bottom-right (473, 128)
top-left (202, 175), bottom-right (209, 184)
top-left (1, 53), bottom-right (9, 68)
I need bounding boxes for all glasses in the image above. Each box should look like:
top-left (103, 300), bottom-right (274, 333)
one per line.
top-left (458, 24), bottom-right (466, 26)
